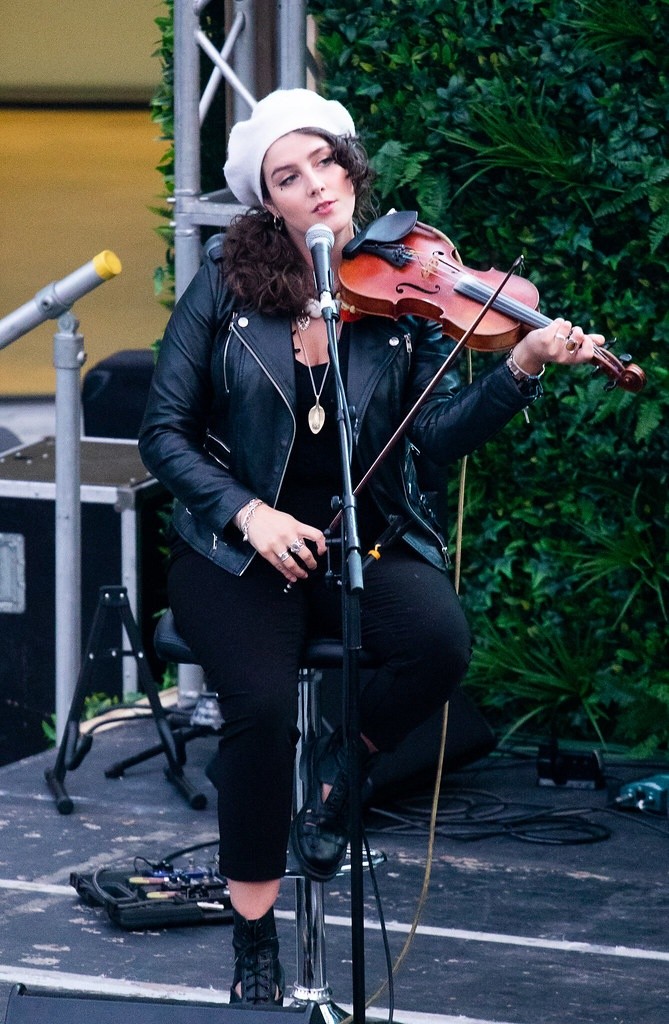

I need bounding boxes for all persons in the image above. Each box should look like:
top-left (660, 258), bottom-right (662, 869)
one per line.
top-left (137, 88), bottom-right (606, 1008)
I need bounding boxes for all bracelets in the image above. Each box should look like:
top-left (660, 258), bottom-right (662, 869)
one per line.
top-left (506, 349), bottom-right (546, 379)
top-left (236, 498), bottom-right (263, 542)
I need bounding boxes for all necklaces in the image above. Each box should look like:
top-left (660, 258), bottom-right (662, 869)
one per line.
top-left (296, 278), bottom-right (339, 331)
top-left (295, 319), bottom-right (344, 435)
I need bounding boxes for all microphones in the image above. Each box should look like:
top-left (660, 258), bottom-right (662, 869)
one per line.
top-left (305, 223), bottom-right (335, 322)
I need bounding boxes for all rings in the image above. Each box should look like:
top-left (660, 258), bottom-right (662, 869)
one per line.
top-left (567, 339), bottom-right (577, 354)
top-left (555, 333), bottom-right (565, 341)
top-left (279, 551), bottom-right (291, 562)
top-left (290, 537), bottom-right (305, 553)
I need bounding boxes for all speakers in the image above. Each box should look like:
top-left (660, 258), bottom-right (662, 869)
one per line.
top-left (3, 983), bottom-right (327, 1024)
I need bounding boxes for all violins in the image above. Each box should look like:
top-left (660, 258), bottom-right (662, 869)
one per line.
top-left (337, 208), bottom-right (650, 394)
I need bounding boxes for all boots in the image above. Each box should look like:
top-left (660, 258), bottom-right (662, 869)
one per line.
top-left (290, 720), bottom-right (387, 882)
top-left (228, 904), bottom-right (286, 1010)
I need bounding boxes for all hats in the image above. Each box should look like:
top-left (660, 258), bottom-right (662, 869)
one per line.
top-left (224, 88), bottom-right (356, 213)
